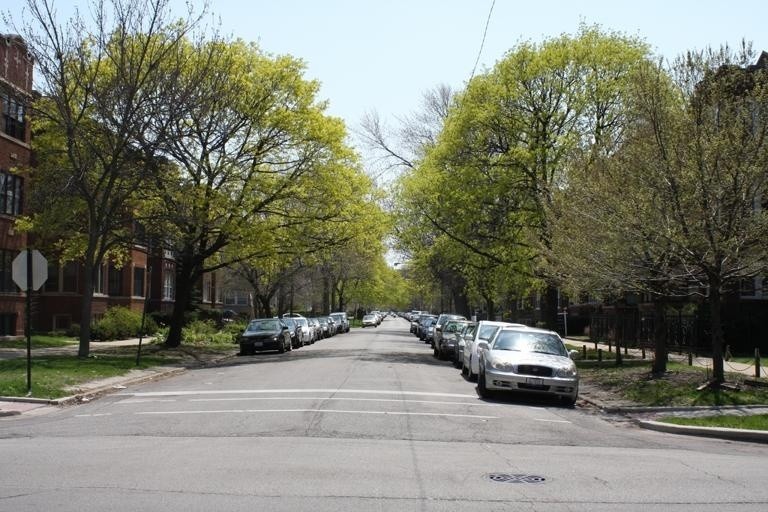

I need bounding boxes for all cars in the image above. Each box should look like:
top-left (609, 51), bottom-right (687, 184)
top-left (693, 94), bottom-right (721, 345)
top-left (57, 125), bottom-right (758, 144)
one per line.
top-left (398, 310), bottom-right (582, 409)
top-left (236, 309), bottom-right (397, 355)
